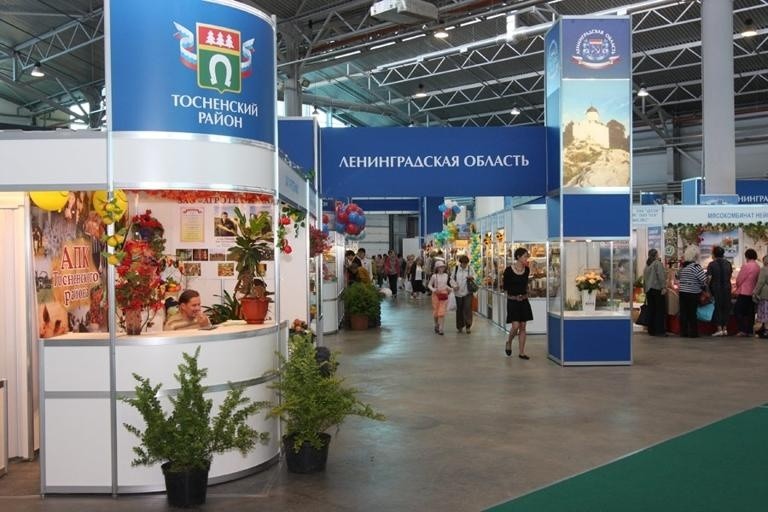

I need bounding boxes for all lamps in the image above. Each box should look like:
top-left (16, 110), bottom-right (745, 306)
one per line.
top-left (742, 20), bottom-right (755, 38)
top-left (416, 80), bottom-right (427, 100)
top-left (510, 103), bottom-right (521, 115)
top-left (639, 83), bottom-right (649, 96)
top-left (433, 19), bottom-right (447, 39)
top-left (30, 61), bottom-right (46, 77)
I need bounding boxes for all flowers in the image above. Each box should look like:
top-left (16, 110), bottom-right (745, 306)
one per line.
top-left (576, 272), bottom-right (603, 293)
top-left (115, 210), bottom-right (170, 311)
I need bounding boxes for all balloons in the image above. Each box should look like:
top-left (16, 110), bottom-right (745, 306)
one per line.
top-left (469, 232), bottom-right (482, 285)
top-left (322, 200), bottom-right (366, 240)
top-left (30, 191), bottom-right (70, 212)
top-left (433, 199), bottom-right (461, 248)
top-left (93, 189), bottom-right (128, 224)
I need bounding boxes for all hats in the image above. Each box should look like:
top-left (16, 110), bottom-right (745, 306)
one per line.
top-left (434, 261), bottom-right (448, 271)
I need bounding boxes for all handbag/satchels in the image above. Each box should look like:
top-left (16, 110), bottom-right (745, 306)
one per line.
top-left (438, 293), bottom-right (449, 301)
top-left (465, 279), bottom-right (478, 292)
top-left (635, 304), bottom-right (650, 326)
top-left (696, 289), bottom-right (715, 322)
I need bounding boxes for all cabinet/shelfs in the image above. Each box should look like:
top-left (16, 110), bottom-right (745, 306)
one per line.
top-left (450, 209), bottom-right (546, 334)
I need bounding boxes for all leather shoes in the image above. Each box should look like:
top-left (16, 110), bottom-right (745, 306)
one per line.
top-left (506, 341), bottom-right (511, 356)
top-left (712, 328), bottom-right (728, 336)
top-left (519, 354), bottom-right (529, 359)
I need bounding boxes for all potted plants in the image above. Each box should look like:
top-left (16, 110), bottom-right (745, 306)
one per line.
top-left (263, 332), bottom-right (387, 474)
top-left (119, 345), bottom-right (271, 509)
top-left (224, 208), bottom-right (275, 324)
top-left (341, 282), bottom-right (385, 329)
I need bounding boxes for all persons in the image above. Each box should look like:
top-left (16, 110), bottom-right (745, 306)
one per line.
top-left (752, 254), bottom-right (768, 338)
top-left (87, 287), bottom-right (105, 328)
top-left (706, 246), bottom-right (733, 337)
top-left (734, 248), bottom-right (760, 337)
top-left (449, 255), bottom-right (477, 334)
top-left (643, 248), bottom-right (668, 336)
top-left (678, 245), bottom-right (705, 337)
top-left (163, 289), bottom-right (213, 332)
top-left (215, 212), bottom-right (235, 236)
top-left (427, 261), bottom-right (457, 336)
top-left (345, 244), bottom-right (433, 299)
top-left (502, 247), bottom-right (534, 359)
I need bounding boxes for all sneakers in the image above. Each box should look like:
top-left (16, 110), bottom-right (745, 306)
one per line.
top-left (434, 325), bottom-right (471, 335)
top-left (736, 329), bottom-right (768, 337)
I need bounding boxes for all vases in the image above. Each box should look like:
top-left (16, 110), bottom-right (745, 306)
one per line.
top-left (125, 308), bottom-right (140, 335)
top-left (581, 291), bottom-right (596, 311)
